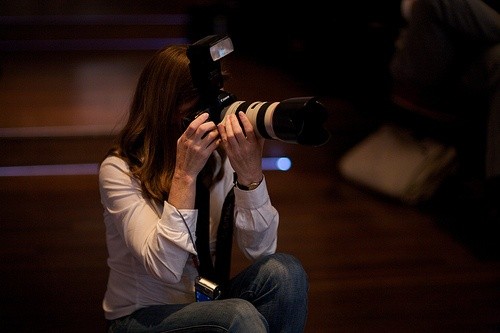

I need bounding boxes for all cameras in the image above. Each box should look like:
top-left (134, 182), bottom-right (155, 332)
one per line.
top-left (182, 34), bottom-right (326, 144)
top-left (195, 277), bottom-right (221, 302)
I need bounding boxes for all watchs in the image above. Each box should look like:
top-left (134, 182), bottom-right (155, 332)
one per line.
top-left (235, 180), bottom-right (260, 189)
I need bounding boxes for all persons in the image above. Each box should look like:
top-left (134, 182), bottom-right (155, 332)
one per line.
top-left (371, 0), bottom-right (500, 264)
top-left (98, 35), bottom-right (310, 333)
